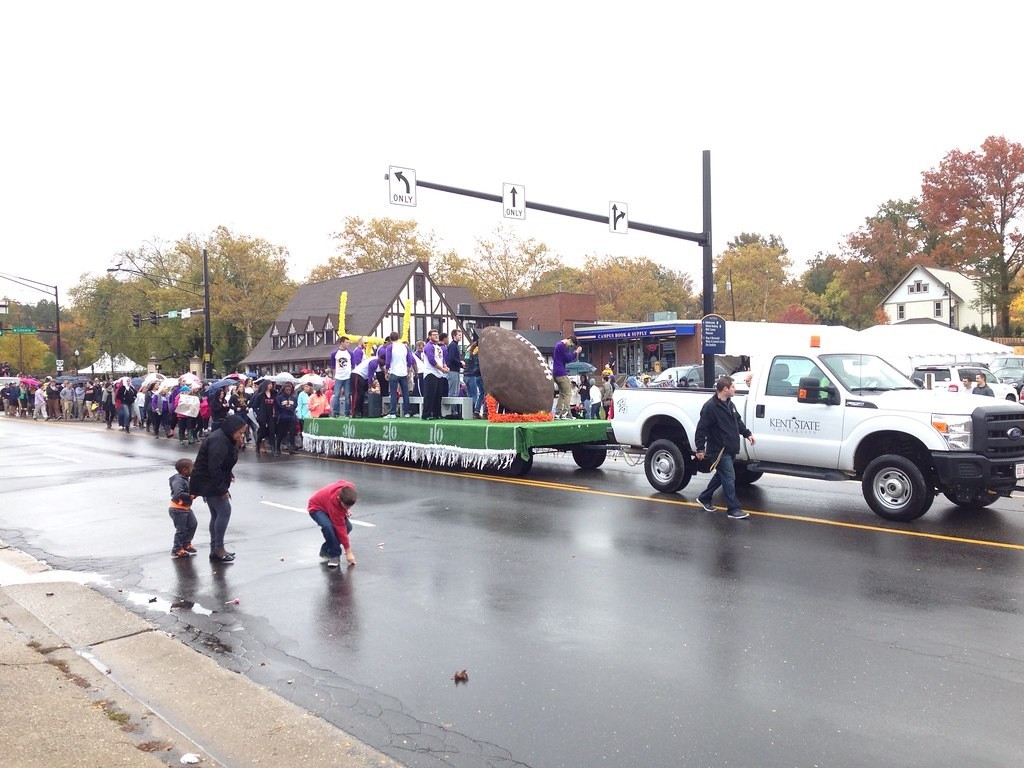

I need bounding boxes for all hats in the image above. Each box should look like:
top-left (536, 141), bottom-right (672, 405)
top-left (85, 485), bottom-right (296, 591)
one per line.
top-left (608, 375), bottom-right (614, 379)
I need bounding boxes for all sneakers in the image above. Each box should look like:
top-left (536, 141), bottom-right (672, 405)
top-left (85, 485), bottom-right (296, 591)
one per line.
top-left (183, 544), bottom-right (196, 553)
top-left (172, 548), bottom-right (191, 557)
top-left (727, 511), bottom-right (750, 519)
top-left (696, 495), bottom-right (717, 512)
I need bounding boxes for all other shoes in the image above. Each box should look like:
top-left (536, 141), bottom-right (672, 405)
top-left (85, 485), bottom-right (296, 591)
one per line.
top-left (273, 452), bottom-right (279, 457)
top-left (346, 414), bottom-right (350, 418)
top-left (413, 413), bottom-right (419, 418)
top-left (405, 413), bottom-right (410, 418)
top-left (331, 413), bottom-right (339, 419)
top-left (554, 413), bottom-right (576, 421)
top-left (425, 416), bottom-right (433, 419)
top-left (328, 556), bottom-right (339, 567)
top-left (383, 414), bottom-right (397, 419)
top-left (289, 450), bottom-right (300, 454)
top-left (5, 412), bottom-right (106, 423)
top-left (256, 446), bottom-right (261, 454)
top-left (320, 551), bottom-right (332, 560)
top-left (209, 549), bottom-right (236, 561)
top-left (107, 422), bottom-right (204, 445)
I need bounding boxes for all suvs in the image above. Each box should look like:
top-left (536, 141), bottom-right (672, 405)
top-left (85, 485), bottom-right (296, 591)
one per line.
top-left (652, 362), bottom-right (731, 391)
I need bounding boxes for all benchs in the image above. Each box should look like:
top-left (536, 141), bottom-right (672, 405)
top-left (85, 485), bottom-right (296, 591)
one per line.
top-left (381, 396), bottom-right (474, 420)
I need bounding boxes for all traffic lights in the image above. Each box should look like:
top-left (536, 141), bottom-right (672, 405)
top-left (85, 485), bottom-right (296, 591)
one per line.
top-left (0, 320), bottom-right (3, 336)
top-left (132, 312), bottom-right (141, 329)
top-left (149, 309), bottom-right (158, 326)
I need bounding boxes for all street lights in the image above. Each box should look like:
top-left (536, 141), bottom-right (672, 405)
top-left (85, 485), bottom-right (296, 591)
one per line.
top-left (97, 339), bottom-right (115, 380)
top-left (106, 249), bottom-right (213, 380)
top-left (978, 264), bottom-right (994, 342)
top-left (941, 281), bottom-right (953, 328)
top-left (73, 349), bottom-right (79, 380)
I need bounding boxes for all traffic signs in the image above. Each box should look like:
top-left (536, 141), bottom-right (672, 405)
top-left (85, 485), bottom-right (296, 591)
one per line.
top-left (388, 164), bottom-right (417, 208)
top-left (502, 182), bottom-right (527, 221)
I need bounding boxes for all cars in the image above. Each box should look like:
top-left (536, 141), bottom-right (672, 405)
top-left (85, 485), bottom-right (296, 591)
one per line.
top-left (907, 355), bottom-right (1024, 405)
top-left (713, 370), bottom-right (752, 391)
top-left (784, 372), bottom-right (812, 386)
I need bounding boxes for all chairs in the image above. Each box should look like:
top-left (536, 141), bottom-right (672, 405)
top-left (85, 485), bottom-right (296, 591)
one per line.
top-left (768, 363), bottom-right (793, 398)
top-left (811, 366), bottom-right (835, 398)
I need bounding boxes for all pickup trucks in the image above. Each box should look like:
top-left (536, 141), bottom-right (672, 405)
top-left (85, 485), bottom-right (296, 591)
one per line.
top-left (610, 330), bottom-right (1023, 523)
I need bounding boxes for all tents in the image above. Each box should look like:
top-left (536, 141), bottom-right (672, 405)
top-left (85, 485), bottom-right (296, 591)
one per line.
top-left (78, 351), bottom-right (147, 374)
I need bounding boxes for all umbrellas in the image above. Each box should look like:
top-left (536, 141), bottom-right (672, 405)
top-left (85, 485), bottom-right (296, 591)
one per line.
top-left (566, 362), bottom-right (597, 385)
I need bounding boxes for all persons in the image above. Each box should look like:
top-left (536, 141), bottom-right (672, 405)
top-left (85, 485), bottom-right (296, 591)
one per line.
top-left (570, 363), bottom-right (638, 420)
top-left (676, 376), bottom-right (689, 387)
top-left (190, 414), bottom-right (248, 561)
top-left (0, 361), bottom-right (336, 456)
top-left (307, 480), bottom-right (357, 566)
top-left (731, 367), bottom-right (739, 375)
top-left (168, 458), bottom-right (197, 557)
top-left (608, 352), bottom-right (615, 374)
top-left (554, 335), bottom-right (582, 420)
top-left (818, 357), bottom-right (873, 399)
top-left (695, 377), bottom-right (756, 518)
top-left (959, 373), bottom-right (995, 398)
top-left (643, 375), bottom-right (651, 385)
top-left (330, 329), bottom-right (510, 422)
